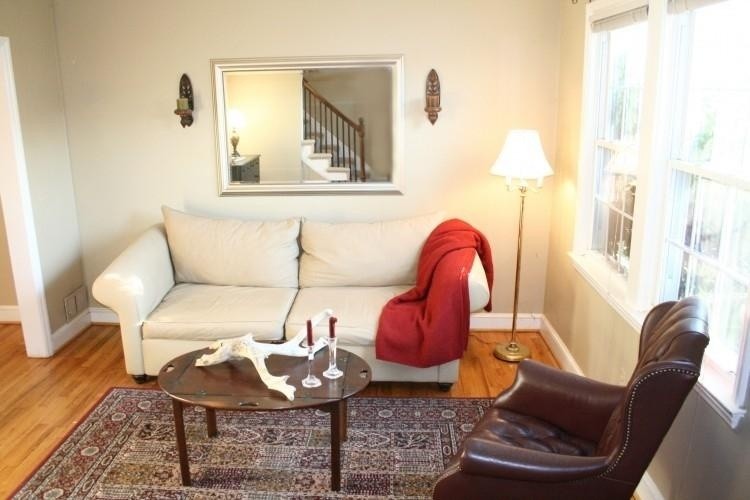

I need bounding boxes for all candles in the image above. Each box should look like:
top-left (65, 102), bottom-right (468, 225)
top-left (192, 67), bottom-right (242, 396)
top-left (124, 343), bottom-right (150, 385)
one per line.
top-left (330, 316), bottom-right (337, 338)
top-left (307, 319), bottom-right (313, 346)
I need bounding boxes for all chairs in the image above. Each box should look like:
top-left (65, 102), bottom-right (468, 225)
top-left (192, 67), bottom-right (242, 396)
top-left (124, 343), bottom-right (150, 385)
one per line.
top-left (434, 295), bottom-right (709, 500)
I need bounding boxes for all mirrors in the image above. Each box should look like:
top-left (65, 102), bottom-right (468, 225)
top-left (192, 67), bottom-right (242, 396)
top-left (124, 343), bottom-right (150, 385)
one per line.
top-left (210, 53), bottom-right (404, 196)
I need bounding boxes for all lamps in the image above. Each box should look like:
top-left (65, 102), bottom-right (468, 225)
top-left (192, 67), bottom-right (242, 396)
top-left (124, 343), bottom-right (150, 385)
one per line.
top-left (488, 127), bottom-right (556, 364)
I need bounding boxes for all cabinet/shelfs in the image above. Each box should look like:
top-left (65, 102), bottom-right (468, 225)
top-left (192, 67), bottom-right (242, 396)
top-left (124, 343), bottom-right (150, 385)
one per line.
top-left (229, 153), bottom-right (260, 183)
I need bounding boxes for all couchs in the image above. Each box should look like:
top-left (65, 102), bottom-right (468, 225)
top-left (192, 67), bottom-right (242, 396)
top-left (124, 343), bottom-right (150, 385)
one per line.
top-left (91, 205), bottom-right (492, 394)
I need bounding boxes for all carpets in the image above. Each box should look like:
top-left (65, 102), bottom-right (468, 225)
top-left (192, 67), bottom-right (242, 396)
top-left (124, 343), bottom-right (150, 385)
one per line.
top-left (7, 386), bottom-right (500, 500)
